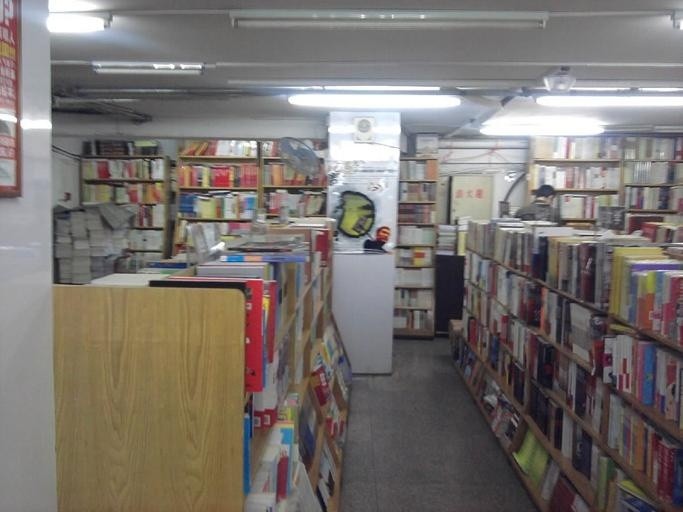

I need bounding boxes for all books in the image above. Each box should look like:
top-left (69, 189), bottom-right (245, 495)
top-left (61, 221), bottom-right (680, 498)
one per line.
top-left (447, 135), bottom-right (682, 511)
top-left (392, 157), bottom-right (456, 332)
top-left (55, 136), bottom-right (352, 512)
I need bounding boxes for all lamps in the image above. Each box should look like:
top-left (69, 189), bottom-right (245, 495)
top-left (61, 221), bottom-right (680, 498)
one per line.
top-left (90, 60), bottom-right (205, 77)
top-left (228, 9), bottom-right (550, 31)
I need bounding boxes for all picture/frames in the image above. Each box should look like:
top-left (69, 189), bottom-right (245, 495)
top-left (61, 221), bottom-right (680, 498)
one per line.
top-left (0, 0), bottom-right (23, 199)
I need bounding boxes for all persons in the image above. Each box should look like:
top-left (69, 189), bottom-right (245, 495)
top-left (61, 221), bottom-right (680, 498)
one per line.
top-left (363, 225), bottom-right (391, 252)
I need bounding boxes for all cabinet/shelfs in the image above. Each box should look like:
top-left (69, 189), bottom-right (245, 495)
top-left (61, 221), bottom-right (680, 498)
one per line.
top-left (77, 155), bottom-right (326, 259)
top-left (393, 157), bottom-right (439, 340)
top-left (52, 216), bottom-right (354, 512)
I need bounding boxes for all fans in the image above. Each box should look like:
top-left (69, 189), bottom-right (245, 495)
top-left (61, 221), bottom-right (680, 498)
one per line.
top-left (276, 136), bottom-right (327, 177)
